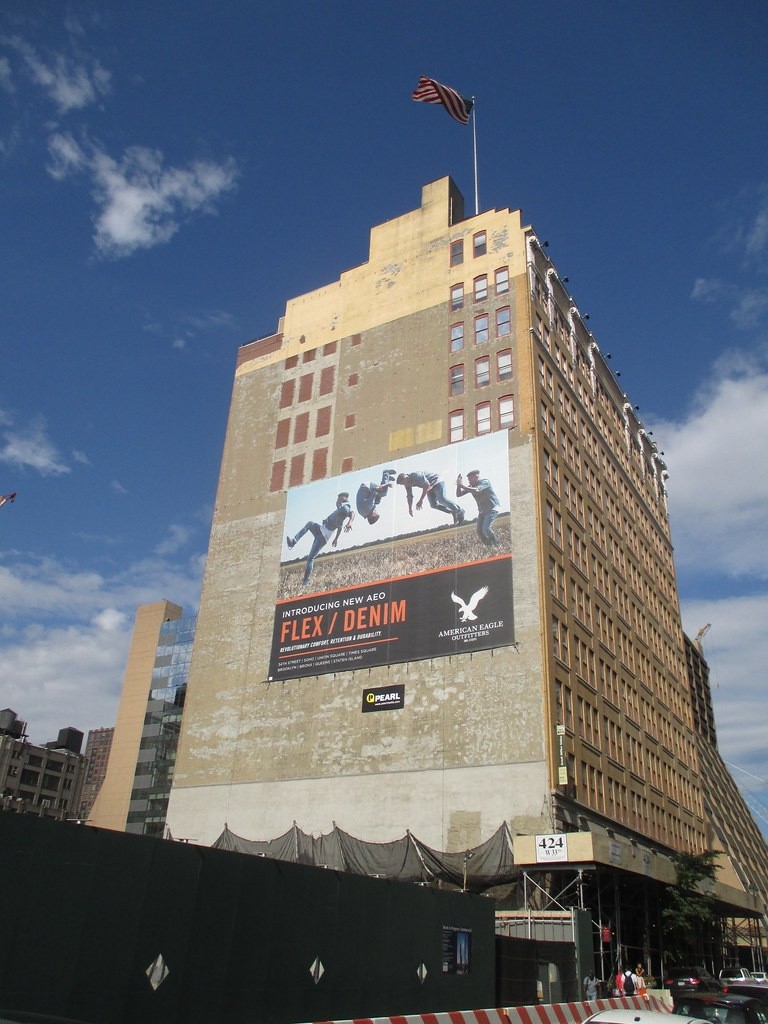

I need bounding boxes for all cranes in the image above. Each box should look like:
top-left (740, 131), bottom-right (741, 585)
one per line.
top-left (695, 623), bottom-right (719, 688)
top-left (0, 492), bottom-right (16, 508)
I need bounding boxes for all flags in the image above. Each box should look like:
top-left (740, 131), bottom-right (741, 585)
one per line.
top-left (411, 72), bottom-right (473, 128)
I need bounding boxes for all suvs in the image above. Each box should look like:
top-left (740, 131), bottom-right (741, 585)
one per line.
top-left (719, 967), bottom-right (756, 988)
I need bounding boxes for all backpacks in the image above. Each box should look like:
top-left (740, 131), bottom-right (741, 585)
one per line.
top-left (623, 972), bottom-right (634, 993)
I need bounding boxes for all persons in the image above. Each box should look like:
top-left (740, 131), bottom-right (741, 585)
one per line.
top-left (455, 469), bottom-right (502, 555)
top-left (616, 970), bottom-right (628, 995)
top-left (635, 962), bottom-right (646, 990)
top-left (287, 492), bottom-right (356, 588)
top-left (397, 471), bottom-right (466, 533)
top-left (356, 469), bottom-right (396, 526)
top-left (584, 968), bottom-right (601, 1002)
top-left (621, 965), bottom-right (638, 997)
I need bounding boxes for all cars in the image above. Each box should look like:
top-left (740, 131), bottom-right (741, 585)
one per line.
top-left (673, 993), bottom-right (768, 1024)
top-left (722, 983), bottom-right (768, 1004)
top-left (750, 971), bottom-right (768, 983)
top-left (582, 1008), bottom-right (714, 1024)
top-left (664, 966), bottom-right (721, 996)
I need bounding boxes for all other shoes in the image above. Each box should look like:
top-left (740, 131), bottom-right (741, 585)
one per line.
top-left (286, 536), bottom-right (295, 549)
top-left (453, 509), bottom-right (465, 524)
top-left (303, 579), bottom-right (310, 587)
top-left (384, 469), bottom-right (396, 481)
